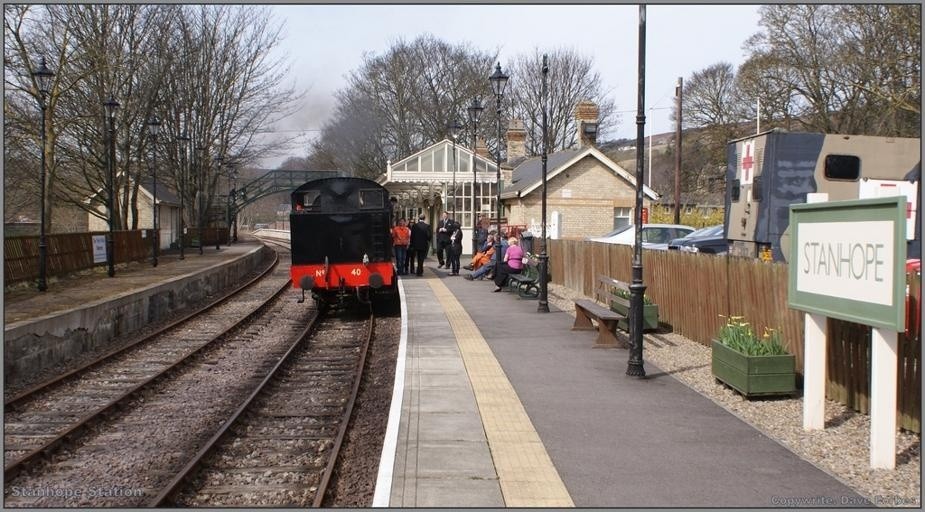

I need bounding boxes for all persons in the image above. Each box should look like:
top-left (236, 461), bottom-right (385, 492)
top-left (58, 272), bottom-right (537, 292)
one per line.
top-left (462, 212), bottom-right (526, 293)
top-left (391, 213), bottom-right (432, 277)
top-left (448, 222), bottom-right (463, 276)
top-left (436, 212), bottom-right (453, 269)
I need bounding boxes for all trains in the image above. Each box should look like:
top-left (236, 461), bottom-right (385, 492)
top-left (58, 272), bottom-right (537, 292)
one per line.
top-left (288, 176), bottom-right (398, 312)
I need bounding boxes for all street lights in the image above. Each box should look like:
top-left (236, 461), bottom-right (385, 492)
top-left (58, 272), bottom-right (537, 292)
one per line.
top-left (176, 133), bottom-right (190, 259)
top-left (103, 91), bottom-right (120, 275)
top-left (468, 95), bottom-right (483, 257)
top-left (215, 152), bottom-right (223, 249)
top-left (195, 142), bottom-right (207, 254)
top-left (488, 62), bottom-right (509, 265)
top-left (34, 56), bottom-right (55, 290)
top-left (226, 162), bottom-right (240, 245)
top-left (146, 115), bottom-right (162, 266)
top-left (447, 117), bottom-right (462, 221)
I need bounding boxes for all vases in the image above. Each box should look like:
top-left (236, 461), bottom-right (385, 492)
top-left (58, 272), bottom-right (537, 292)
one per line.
top-left (610, 300), bottom-right (659, 333)
top-left (711, 339), bottom-right (797, 400)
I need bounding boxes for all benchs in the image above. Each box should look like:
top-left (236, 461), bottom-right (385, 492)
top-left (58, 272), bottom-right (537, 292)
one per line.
top-left (507, 259), bottom-right (540, 298)
top-left (571, 274), bottom-right (631, 348)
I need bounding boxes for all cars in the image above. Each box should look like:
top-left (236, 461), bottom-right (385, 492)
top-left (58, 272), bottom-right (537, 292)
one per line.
top-left (587, 224), bottom-right (731, 255)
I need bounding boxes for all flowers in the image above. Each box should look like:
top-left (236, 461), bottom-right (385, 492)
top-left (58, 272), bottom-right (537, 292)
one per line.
top-left (611, 286), bottom-right (652, 305)
top-left (718, 314), bottom-right (792, 356)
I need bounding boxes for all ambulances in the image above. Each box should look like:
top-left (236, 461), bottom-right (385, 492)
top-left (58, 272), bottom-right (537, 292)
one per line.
top-left (725, 131), bottom-right (921, 266)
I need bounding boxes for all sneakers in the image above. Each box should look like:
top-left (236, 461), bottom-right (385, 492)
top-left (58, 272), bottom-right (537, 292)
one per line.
top-left (487, 275), bottom-right (501, 292)
top-left (438, 262), bottom-right (473, 280)
